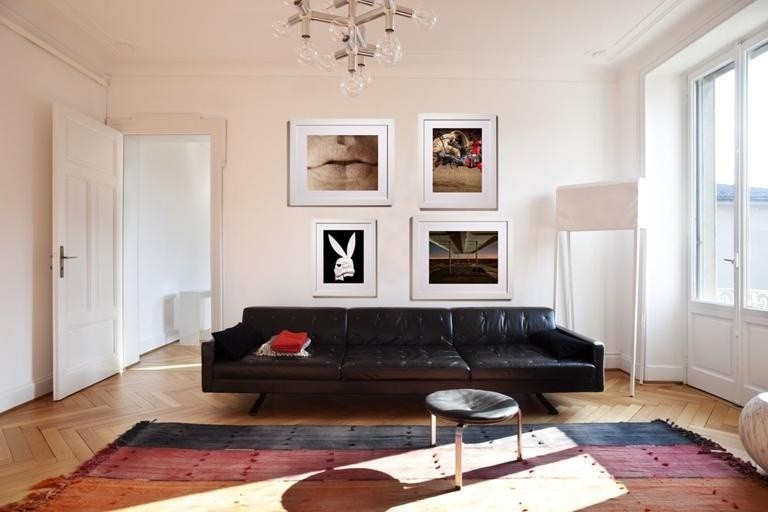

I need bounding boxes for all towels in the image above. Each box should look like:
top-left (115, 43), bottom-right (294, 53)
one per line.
top-left (271, 329), bottom-right (314, 354)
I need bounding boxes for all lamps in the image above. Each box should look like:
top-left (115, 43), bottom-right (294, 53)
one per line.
top-left (268, 0), bottom-right (439, 96)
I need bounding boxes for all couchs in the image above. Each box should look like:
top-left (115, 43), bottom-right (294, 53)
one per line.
top-left (199, 304), bottom-right (607, 418)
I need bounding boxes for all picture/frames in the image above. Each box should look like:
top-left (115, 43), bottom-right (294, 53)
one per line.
top-left (312, 219), bottom-right (380, 300)
top-left (411, 216), bottom-right (514, 304)
top-left (416, 114), bottom-right (500, 213)
top-left (288, 118), bottom-right (394, 208)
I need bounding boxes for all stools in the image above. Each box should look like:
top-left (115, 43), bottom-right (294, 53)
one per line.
top-left (426, 387), bottom-right (522, 489)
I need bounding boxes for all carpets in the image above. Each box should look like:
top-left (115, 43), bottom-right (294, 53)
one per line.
top-left (4, 418), bottom-right (767, 512)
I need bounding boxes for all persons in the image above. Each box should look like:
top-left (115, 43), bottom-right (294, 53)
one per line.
top-left (436, 132), bottom-right (482, 168)
